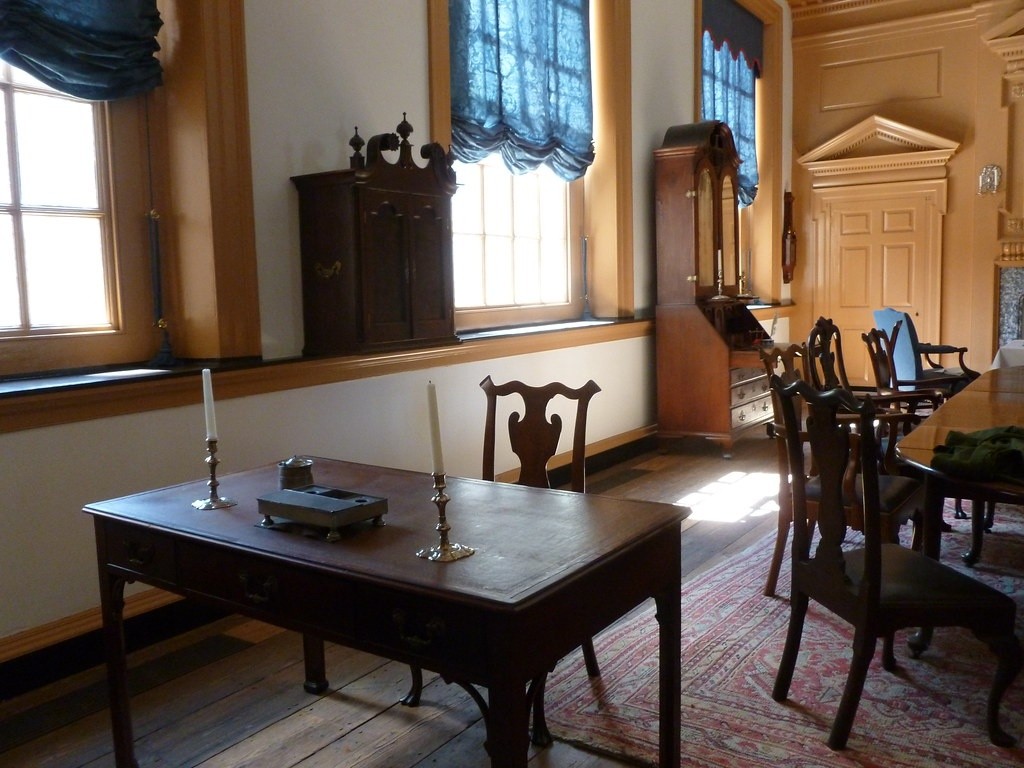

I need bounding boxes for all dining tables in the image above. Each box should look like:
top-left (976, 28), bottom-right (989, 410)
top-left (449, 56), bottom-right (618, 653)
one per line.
top-left (893, 366), bottom-right (1024, 659)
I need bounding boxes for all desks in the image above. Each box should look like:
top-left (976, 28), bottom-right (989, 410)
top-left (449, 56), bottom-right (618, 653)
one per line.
top-left (990, 340), bottom-right (1024, 370)
top-left (83, 454), bottom-right (692, 768)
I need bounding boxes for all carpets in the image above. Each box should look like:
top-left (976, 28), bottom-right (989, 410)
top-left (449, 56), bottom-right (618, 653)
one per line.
top-left (527, 497), bottom-right (1024, 768)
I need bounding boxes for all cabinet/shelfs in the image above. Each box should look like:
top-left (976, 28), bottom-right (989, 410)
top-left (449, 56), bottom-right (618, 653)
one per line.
top-left (289, 112), bottom-right (464, 359)
top-left (651, 120), bottom-right (774, 459)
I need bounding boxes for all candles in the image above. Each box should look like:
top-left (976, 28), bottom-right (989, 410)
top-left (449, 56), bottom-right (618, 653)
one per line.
top-left (202, 368), bottom-right (218, 440)
top-left (718, 250), bottom-right (722, 278)
top-left (426, 380), bottom-right (445, 474)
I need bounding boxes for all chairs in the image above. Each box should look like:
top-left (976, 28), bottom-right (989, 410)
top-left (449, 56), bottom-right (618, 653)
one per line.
top-left (756, 307), bottom-right (1024, 750)
top-left (400, 374), bottom-right (602, 752)
